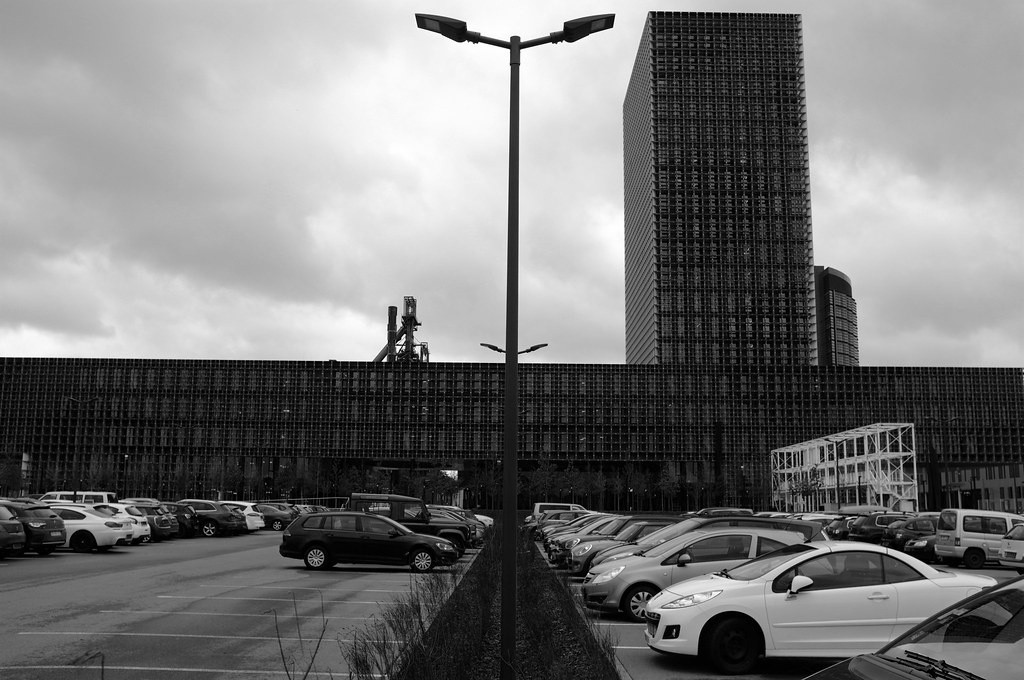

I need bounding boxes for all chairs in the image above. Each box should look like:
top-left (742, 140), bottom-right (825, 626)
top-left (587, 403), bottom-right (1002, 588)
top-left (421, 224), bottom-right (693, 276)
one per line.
top-left (725, 540), bottom-right (745, 558)
top-left (835, 556), bottom-right (870, 588)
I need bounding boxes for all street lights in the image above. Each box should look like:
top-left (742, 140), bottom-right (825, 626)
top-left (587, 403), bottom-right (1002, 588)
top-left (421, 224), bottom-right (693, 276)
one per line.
top-left (413, 9), bottom-right (622, 680)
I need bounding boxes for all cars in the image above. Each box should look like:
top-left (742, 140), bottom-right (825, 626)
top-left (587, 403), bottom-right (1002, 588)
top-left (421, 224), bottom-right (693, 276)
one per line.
top-left (644, 538), bottom-right (1000, 675)
top-left (808, 572), bottom-right (1024, 680)
top-left (0, 499), bottom-right (67, 555)
top-left (278, 511), bottom-right (459, 574)
top-left (39, 488), bottom-right (495, 554)
top-left (524, 496), bottom-right (1023, 624)
top-left (0, 502), bottom-right (27, 560)
